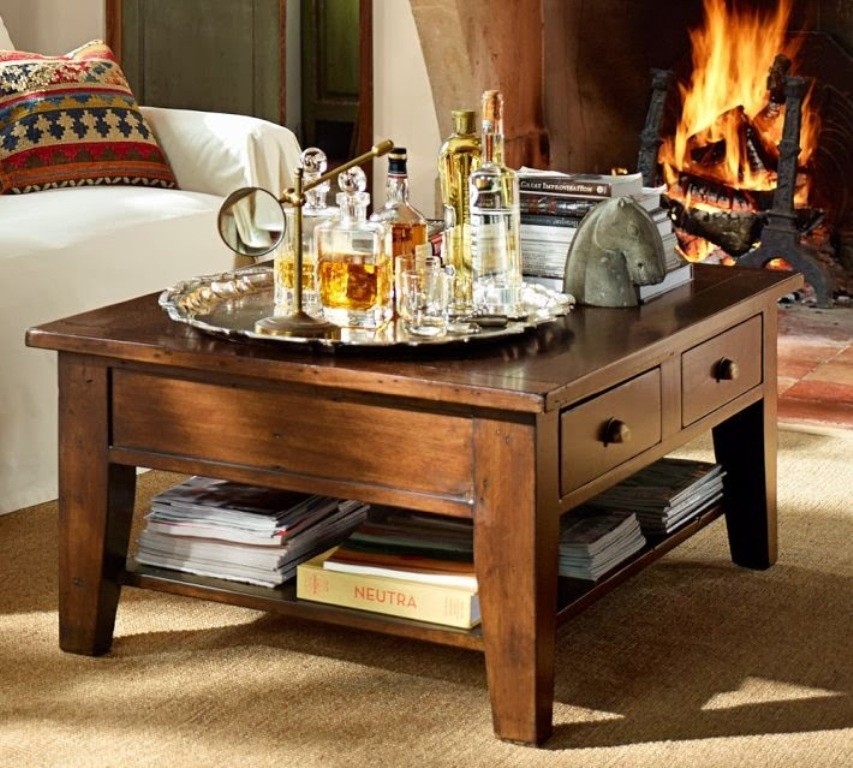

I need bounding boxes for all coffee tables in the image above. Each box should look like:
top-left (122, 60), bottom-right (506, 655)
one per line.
top-left (35, 262), bottom-right (805, 750)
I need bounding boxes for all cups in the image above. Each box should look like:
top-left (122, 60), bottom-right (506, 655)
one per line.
top-left (395, 255), bottom-right (442, 314)
top-left (402, 270), bottom-right (449, 337)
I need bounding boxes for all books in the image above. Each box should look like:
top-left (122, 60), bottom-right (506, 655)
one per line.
top-left (517, 164), bottom-right (693, 306)
top-left (133, 474), bottom-right (475, 590)
top-left (559, 457), bottom-right (728, 582)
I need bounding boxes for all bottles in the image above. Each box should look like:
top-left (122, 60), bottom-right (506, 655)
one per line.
top-left (273, 90), bottom-right (526, 345)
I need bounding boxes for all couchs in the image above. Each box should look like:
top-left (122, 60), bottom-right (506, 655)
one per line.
top-left (0, 13), bottom-right (303, 518)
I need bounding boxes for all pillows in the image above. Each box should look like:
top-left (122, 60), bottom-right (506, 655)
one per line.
top-left (0, 40), bottom-right (180, 198)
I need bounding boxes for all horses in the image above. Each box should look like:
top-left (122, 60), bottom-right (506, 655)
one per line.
top-left (560, 192), bottom-right (666, 309)
